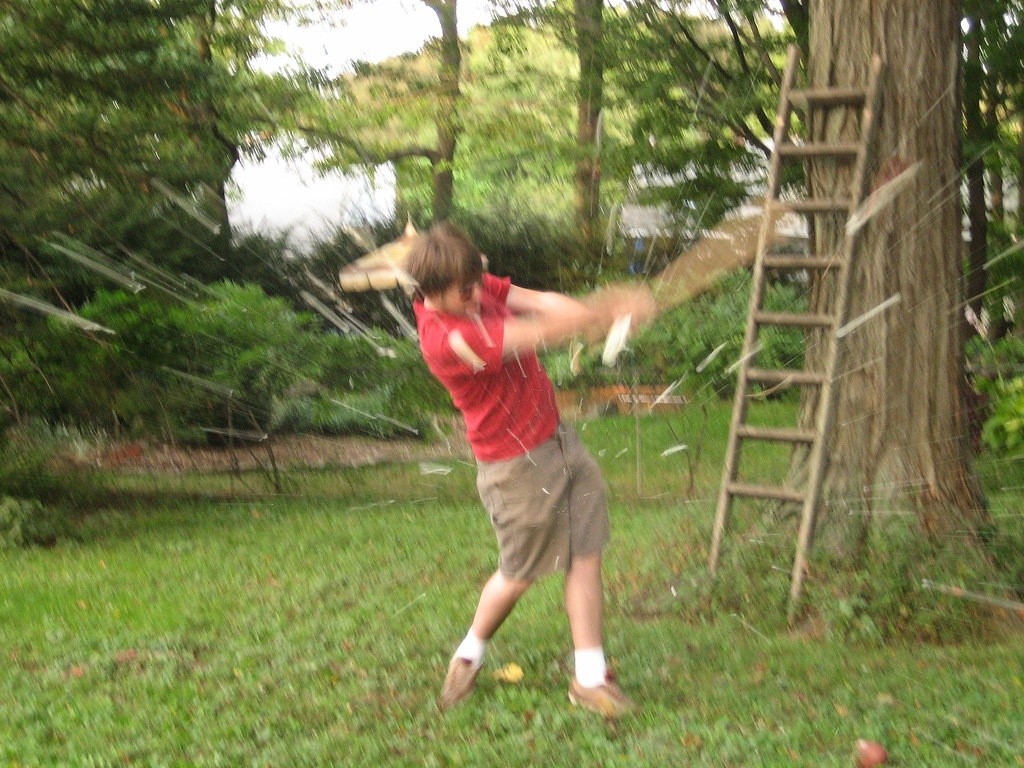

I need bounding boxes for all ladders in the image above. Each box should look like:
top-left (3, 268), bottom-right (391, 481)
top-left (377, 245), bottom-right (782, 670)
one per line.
top-left (701, 42), bottom-right (885, 627)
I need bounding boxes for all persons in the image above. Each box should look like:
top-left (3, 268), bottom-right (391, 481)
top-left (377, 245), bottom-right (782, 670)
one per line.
top-left (412, 218), bottom-right (663, 720)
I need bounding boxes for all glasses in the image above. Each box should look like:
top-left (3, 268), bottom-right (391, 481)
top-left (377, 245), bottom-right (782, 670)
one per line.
top-left (440, 274), bottom-right (483, 303)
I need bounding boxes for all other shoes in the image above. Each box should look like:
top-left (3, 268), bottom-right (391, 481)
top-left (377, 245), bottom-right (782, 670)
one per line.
top-left (440, 651), bottom-right (484, 706)
top-left (568, 671), bottom-right (639, 719)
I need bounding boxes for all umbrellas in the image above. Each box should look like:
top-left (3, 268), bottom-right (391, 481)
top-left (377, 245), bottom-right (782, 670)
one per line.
top-left (337, 222), bottom-right (492, 298)
